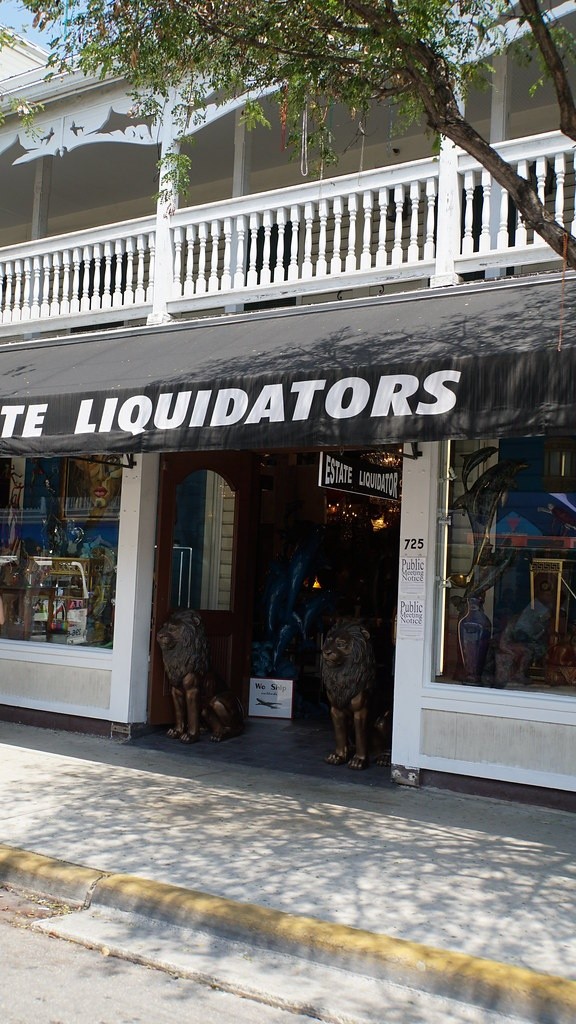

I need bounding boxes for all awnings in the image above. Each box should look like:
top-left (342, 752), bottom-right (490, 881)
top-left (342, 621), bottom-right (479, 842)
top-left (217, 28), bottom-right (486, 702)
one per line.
top-left (0, 280), bottom-right (575, 458)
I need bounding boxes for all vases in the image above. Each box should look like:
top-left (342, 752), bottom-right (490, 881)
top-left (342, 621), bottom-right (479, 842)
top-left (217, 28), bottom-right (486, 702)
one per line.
top-left (456, 596), bottom-right (492, 684)
top-left (524, 555), bottom-right (576, 681)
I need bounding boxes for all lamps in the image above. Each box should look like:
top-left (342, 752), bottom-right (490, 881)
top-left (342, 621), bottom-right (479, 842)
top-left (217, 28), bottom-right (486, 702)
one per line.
top-left (530, 160), bottom-right (555, 197)
top-left (257, 453), bottom-right (278, 468)
top-left (261, 474), bottom-right (274, 493)
top-left (386, 189), bottom-right (410, 222)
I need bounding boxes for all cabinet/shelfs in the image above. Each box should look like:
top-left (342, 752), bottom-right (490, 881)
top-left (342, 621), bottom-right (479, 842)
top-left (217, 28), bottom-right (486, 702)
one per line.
top-left (0, 554), bottom-right (104, 641)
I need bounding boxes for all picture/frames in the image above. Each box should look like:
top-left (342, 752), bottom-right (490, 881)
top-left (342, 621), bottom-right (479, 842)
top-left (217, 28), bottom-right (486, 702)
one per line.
top-left (61, 456), bottom-right (122, 522)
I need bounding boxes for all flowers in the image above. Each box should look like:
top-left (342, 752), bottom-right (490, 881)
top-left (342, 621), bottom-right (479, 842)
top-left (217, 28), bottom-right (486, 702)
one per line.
top-left (546, 507), bottom-right (576, 555)
